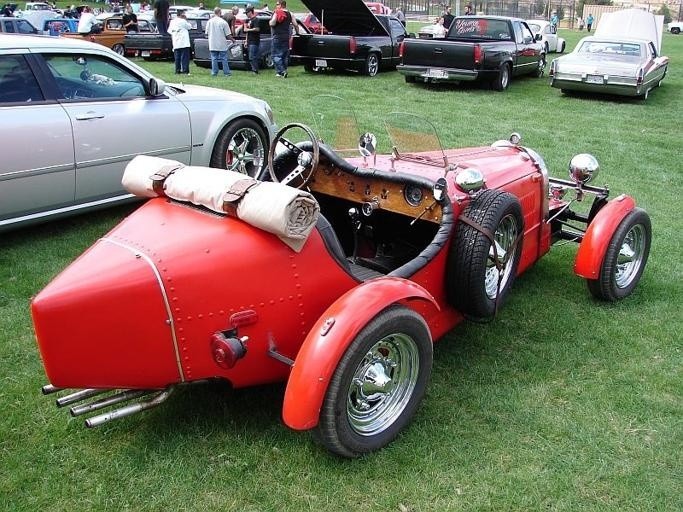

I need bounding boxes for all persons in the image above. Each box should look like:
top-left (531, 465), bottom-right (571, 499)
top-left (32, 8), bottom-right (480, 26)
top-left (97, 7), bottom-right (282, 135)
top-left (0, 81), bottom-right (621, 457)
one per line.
top-left (205, 0), bottom-right (300, 79)
top-left (578, 17), bottom-right (585, 33)
top-left (199, 2), bottom-right (206, 10)
top-left (1, 0), bottom-right (170, 37)
top-left (464, 5), bottom-right (474, 15)
top-left (166, 9), bottom-right (192, 75)
top-left (551, 12), bottom-right (558, 31)
top-left (394, 6), bottom-right (405, 27)
top-left (586, 13), bottom-right (594, 33)
top-left (434, 17), bottom-right (446, 38)
top-left (440, 5), bottom-right (456, 28)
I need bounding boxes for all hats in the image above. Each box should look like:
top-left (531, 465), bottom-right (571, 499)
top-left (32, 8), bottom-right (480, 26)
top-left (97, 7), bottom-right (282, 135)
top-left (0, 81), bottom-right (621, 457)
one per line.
top-left (242, 7), bottom-right (254, 14)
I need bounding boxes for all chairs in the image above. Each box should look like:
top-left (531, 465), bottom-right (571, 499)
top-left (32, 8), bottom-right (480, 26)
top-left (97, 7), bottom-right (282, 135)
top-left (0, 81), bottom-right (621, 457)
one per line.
top-left (313, 210), bottom-right (385, 285)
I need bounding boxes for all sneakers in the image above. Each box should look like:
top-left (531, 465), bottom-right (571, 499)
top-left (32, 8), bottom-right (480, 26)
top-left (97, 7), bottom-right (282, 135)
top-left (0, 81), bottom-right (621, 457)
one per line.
top-left (224, 73), bottom-right (233, 78)
top-left (174, 71), bottom-right (193, 76)
top-left (246, 70), bottom-right (259, 75)
top-left (275, 70), bottom-right (289, 79)
top-left (210, 74), bottom-right (217, 77)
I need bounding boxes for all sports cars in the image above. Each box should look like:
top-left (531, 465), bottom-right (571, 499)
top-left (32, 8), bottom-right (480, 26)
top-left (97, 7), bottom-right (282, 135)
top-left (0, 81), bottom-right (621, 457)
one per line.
top-left (33, 94), bottom-right (655, 461)
